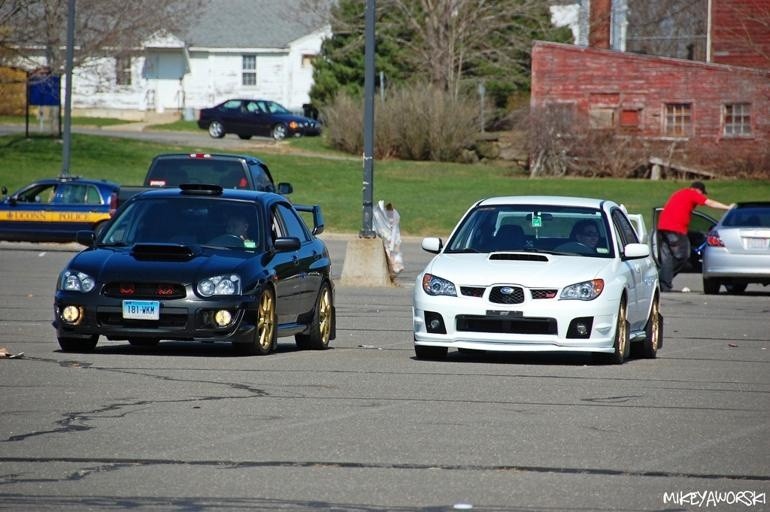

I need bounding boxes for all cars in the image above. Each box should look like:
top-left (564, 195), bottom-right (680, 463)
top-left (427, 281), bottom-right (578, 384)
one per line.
top-left (651, 201), bottom-right (770, 294)
top-left (412, 195), bottom-right (663, 364)
top-left (197, 99), bottom-right (321, 141)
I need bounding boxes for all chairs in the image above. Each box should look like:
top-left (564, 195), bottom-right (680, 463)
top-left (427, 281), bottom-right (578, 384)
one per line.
top-left (492, 225), bottom-right (526, 251)
top-left (167, 214), bottom-right (202, 244)
top-left (569, 219), bottom-right (583, 242)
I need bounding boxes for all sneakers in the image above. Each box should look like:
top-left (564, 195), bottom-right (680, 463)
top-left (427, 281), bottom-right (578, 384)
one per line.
top-left (660, 276), bottom-right (674, 292)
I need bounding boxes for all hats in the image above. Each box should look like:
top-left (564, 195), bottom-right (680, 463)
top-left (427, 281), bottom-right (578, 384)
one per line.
top-left (689, 182), bottom-right (708, 194)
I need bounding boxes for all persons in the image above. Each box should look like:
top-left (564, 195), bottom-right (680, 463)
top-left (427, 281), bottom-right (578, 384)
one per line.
top-left (570, 219), bottom-right (600, 251)
top-left (223, 212), bottom-right (249, 247)
top-left (657, 181), bottom-right (737, 293)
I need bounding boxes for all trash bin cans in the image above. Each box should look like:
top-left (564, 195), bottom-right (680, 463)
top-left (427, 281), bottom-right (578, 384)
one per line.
top-left (372, 200), bottom-right (405, 279)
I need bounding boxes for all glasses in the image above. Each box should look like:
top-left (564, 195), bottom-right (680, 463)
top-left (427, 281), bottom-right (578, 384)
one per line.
top-left (579, 230), bottom-right (600, 236)
